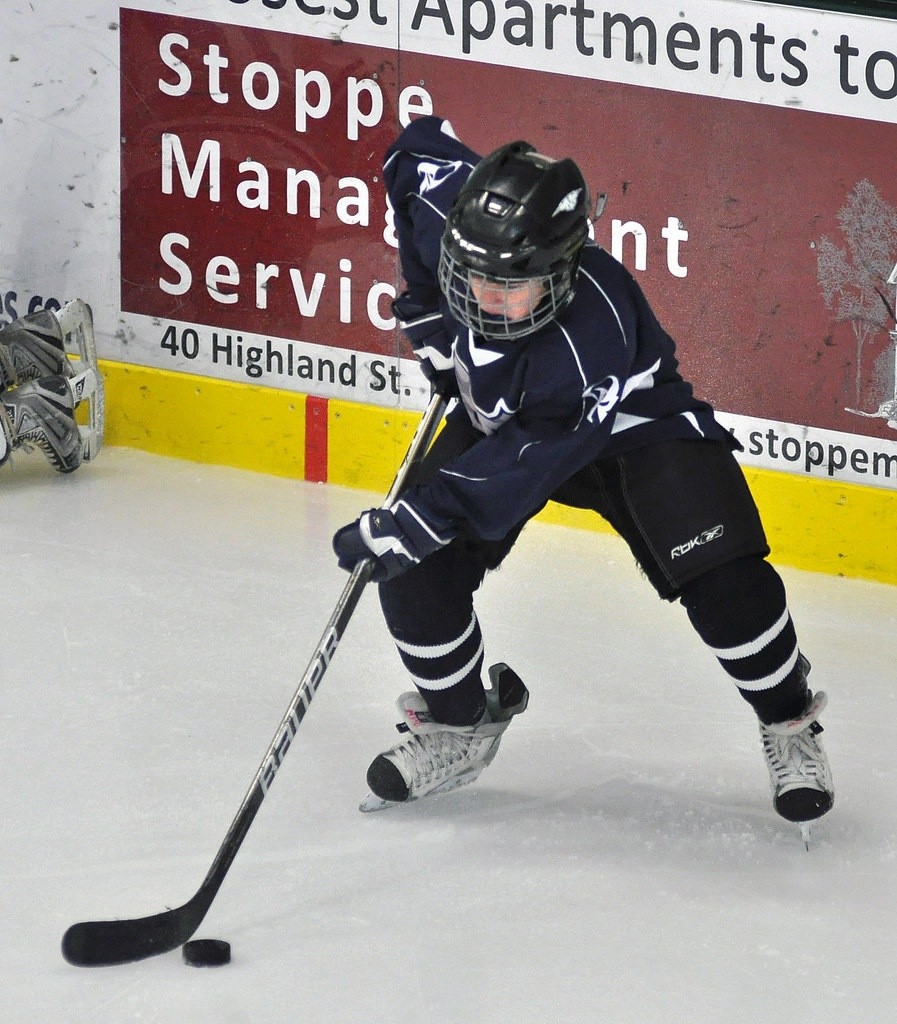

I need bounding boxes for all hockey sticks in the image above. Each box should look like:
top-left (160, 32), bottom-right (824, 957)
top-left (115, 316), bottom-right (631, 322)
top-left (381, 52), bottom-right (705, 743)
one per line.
top-left (59, 384), bottom-right (449, 968)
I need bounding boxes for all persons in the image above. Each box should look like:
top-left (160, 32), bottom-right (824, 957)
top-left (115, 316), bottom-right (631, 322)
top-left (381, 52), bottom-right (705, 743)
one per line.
top-left (0, 298), bottom-right (104, 473)
top-left (331, 114), bottom-right (836, 851)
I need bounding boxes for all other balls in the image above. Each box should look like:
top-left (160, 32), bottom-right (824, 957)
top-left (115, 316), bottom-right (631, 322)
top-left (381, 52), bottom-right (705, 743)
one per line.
top-left (183, 938), bottom-right (231, 967)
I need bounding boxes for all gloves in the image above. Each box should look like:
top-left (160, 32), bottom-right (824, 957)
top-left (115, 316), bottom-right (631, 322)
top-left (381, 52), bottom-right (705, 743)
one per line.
top-left (390, 287), bottom-right (457, 399)
top-left (332, 493), bottom-right (458, 584)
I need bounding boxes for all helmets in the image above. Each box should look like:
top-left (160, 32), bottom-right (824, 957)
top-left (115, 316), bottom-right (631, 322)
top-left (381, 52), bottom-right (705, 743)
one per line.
top-left (437, 140), bottom-right (589, 342)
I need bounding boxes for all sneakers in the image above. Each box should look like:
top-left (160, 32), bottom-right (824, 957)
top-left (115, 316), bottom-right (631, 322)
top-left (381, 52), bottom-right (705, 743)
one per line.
top-left (759, 655), bottom-right (835, 852)
top-left (358, 662), bottom-right (529, 813)
